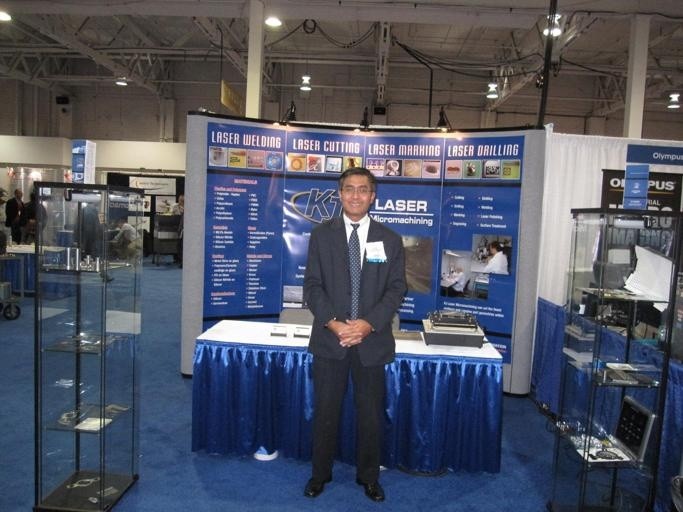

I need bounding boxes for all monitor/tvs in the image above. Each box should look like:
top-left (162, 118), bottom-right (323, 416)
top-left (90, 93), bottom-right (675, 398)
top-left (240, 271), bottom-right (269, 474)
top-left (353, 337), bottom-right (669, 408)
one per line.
top-left (613, 395), bottom-right (656, 463)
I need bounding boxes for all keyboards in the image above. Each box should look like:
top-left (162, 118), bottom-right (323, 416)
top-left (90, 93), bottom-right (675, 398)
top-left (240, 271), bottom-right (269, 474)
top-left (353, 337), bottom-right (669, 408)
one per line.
top-left (599, 367), bottom-right (639, 384)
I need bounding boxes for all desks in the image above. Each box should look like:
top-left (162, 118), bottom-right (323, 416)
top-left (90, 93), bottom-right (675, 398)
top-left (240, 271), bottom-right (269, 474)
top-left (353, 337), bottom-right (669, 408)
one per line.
top-left (0, 246), bottom-right (78, 302)
top-left (189, 318), bottom-right (505, 476)
top-left (531, 298), bottom-right (683, 507)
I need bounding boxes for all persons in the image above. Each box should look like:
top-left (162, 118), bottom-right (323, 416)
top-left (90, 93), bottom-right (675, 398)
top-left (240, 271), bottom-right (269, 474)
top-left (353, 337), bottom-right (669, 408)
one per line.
top-left (110, 217), bottom-right (143, 272)
top-left (175, 193), bottom-right (184, 269)
top-left (302, 167), bottom-right (407, 502)
top-left (481, 240), bottom-right (508, 275)
top-left (447, 266), bottom-right (465, 297)
top-left (72, 201), bottom-right (115, 282)
top-left (3, 188), bottom-right (25, 245)
top-left (18, 192), bottom-right (48, 244)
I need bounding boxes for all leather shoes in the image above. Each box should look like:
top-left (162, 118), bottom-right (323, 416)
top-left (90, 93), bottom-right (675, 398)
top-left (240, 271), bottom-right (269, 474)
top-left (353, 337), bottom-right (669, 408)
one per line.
top-left (353, 474), bottom-right (385, 503)
top-left (304, 472), bottom-right (333, 498)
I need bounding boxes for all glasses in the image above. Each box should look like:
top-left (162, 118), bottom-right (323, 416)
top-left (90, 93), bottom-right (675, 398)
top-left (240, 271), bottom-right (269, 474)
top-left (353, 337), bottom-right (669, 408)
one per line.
top-left (339, 186), bottom-right (376, 196)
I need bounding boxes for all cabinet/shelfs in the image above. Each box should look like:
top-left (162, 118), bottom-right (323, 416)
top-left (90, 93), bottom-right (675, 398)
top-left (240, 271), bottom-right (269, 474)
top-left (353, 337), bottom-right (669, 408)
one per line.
top-left (32, 180), bottom-right (144, 512)
top-left (546, 208), bottom-right (683, 511)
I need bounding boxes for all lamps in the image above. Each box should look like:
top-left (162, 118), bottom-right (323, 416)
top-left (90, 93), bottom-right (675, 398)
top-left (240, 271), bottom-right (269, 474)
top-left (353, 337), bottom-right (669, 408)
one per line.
top-left (486, 82), bottom-right (499, 99)
top-left (299, 73), bottom-right (313, 92)
top-left (666, 93), bottom-right (681, 109)
top-left (435, 105), bottom-right (453, 134)
top-left (359, 105), bottom-right (375, 135)
top-left (542, 13), bottom-right (562, 38)
top-left (278, 100), bottom-right (298, 127)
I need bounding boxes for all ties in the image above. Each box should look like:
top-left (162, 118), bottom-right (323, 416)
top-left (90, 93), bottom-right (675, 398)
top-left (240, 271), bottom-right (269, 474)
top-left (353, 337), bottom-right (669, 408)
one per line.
top-left (347, 224), bottom-right (363, 321)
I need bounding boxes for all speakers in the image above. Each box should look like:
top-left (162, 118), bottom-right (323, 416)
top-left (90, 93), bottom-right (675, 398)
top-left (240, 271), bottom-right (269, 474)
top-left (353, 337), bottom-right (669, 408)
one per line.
top-left (55, 95), bottom-right (69, 105)
top-left (374, 107), bottom-right (385, 115)
